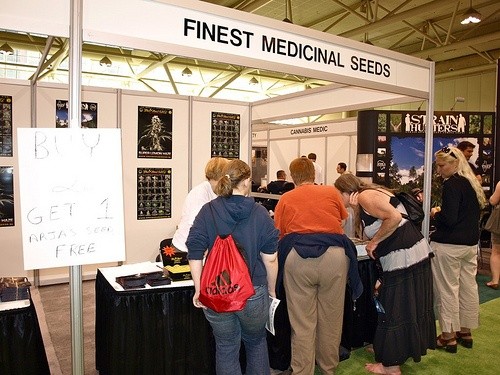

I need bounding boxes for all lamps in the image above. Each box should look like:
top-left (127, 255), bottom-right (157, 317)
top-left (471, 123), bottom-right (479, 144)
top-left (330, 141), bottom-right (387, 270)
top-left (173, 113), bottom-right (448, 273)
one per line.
top-left (460, 0), bottom-right (481, 25)
top-left (182, 66), bottom-right (192, 77)
top-left (99, 52), bottom-right (112, 67)
top-left (0, 41), bottom-right (14, 55)
top-left (450, 96), bottom-right (464, 110)
top-left (249, 75), bottom-right (259, 85)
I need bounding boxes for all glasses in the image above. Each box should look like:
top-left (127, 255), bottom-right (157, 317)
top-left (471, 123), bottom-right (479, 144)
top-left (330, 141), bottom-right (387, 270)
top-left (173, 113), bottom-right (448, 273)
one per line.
top-left (442, 146), bottom-right (457, 159)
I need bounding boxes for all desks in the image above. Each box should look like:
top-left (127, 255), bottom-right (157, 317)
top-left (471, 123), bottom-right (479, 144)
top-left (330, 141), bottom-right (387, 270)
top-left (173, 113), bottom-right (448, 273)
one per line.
top-left (94, 248), bottom-right (374, 375)
top-left (0, 291), bottom-right (51, 375)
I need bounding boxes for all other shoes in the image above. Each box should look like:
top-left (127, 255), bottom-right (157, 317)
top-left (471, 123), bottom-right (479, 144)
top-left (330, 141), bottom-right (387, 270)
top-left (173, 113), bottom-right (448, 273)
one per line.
top-left (485, 282), bottom-right (499, 290)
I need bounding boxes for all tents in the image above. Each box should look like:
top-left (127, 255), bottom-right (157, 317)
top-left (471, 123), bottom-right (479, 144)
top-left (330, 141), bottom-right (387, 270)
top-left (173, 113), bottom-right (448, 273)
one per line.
top-left (0, 0), bottom-right (435, 375)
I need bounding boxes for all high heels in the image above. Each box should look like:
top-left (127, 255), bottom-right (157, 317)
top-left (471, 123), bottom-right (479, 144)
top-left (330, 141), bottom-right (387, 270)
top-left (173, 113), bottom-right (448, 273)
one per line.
top-left (455, 330), bottom-right (473, 349)
top-left (436, 335), bottom-right (458, 353)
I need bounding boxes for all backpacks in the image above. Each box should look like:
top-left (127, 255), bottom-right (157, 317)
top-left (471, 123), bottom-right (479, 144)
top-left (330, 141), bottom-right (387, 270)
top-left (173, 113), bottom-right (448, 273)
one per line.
top-left (362, 190), bottom-right (424, 223)
top-left (198, 203), bottom-right (255, 312)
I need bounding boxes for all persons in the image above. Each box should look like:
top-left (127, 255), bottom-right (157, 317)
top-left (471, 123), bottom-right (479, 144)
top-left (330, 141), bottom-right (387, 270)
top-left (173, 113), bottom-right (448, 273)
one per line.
top-left (308, 153), bottom-right (323, 185)
top-left (186, 159), bottom-right (280, 375)
top-left (274, 159), bottom-right (363, 375)
top-left (170, 157), bottom-right (228, 252)
top-left (457, 140), bottom-right (481, 183)
top-left (410, 187), bottom-right (438, 208)
top-left (333, 174), bottom-right (438, 375)
top-left (337, 162), bottom-right (347, 175)
top-left (253, 171), bottom-right (294, 212)
top-left (485, 180), bottom-right (500, 289)
top-left (430, 147), bottom-right (485, 353)
